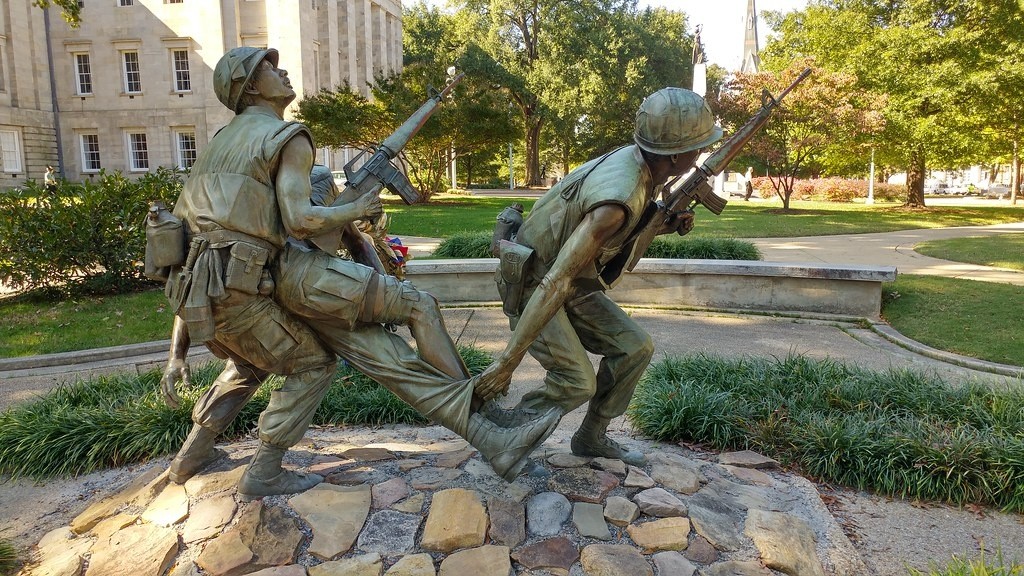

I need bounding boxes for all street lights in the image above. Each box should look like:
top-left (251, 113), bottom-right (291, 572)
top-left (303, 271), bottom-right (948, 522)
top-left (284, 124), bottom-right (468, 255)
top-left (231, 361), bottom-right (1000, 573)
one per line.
top-left (446, 66), bottom-right (456, 189)
top-left (445, 78), bottom-right (452, 190)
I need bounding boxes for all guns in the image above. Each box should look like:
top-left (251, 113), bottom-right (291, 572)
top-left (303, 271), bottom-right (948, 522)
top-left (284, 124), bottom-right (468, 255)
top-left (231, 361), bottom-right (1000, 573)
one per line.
top-left (307, 70), bottom-right (467, 257)
top-left (659, 70), bottom-right (810, 238)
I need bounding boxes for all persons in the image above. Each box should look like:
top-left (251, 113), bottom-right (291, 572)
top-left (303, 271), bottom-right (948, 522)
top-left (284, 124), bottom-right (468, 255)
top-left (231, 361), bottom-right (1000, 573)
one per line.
top-left (156, 125), bottom-right (564, 482)
top-left (744, 165), bottom-right (755, 202)
top-left (144, 46), bottom-right (384, 500)
top-left (44, 166), bottom-right (57, 196)
top-left (475, 82), bottom-right (730, 468)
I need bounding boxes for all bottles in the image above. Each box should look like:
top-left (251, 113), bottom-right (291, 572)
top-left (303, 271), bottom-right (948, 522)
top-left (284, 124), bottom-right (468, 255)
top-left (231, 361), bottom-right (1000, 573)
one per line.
top-left (490, 201), bottom-right (525, 259)
top-left (145, 202), bottom-right (183, 269)
